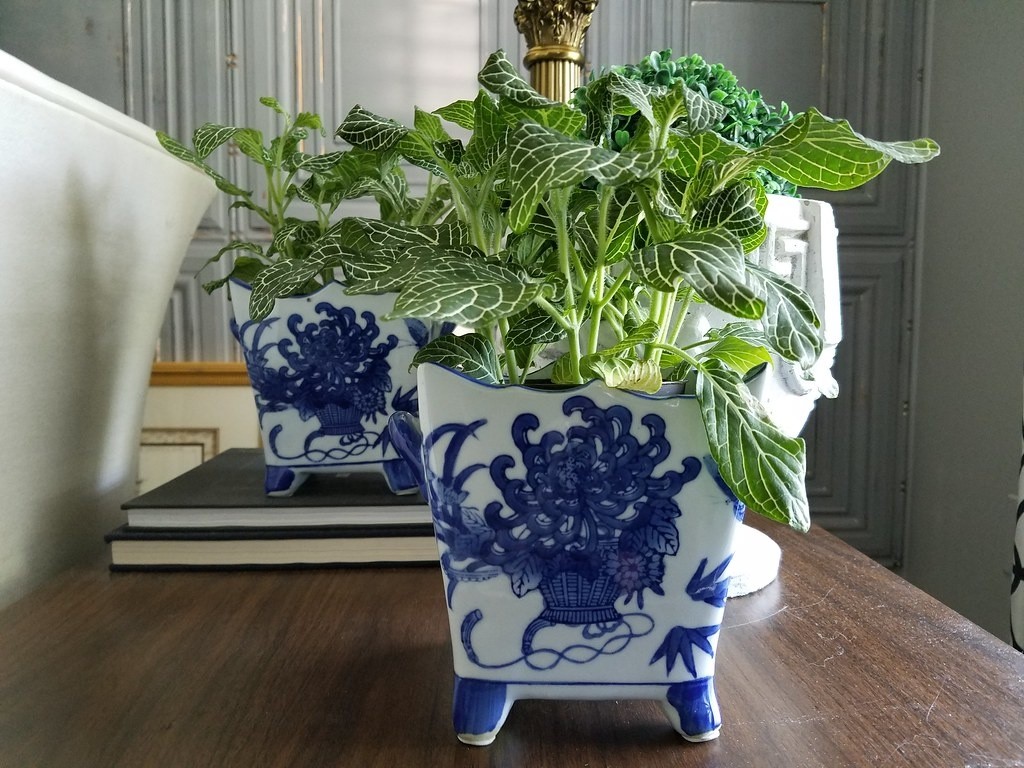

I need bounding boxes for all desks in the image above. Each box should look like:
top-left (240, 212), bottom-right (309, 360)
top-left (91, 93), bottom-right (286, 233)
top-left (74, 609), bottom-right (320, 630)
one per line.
top-left (6, 475), bottom-right (1023, 768)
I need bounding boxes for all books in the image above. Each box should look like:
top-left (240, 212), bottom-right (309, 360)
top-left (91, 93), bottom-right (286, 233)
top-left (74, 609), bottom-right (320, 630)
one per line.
top-left (128, 447), bottom-right (433, 525)
top-left (104, 524), bottom-right (438, 574)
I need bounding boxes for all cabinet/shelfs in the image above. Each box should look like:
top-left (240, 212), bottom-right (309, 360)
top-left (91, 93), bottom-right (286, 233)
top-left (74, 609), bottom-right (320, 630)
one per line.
top-left (138, 380), bottom-right (261, 494)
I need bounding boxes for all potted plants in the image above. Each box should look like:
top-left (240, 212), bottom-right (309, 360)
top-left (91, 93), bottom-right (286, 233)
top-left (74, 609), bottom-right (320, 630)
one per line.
top-left (150, 55), bottom-right (939, 746)
